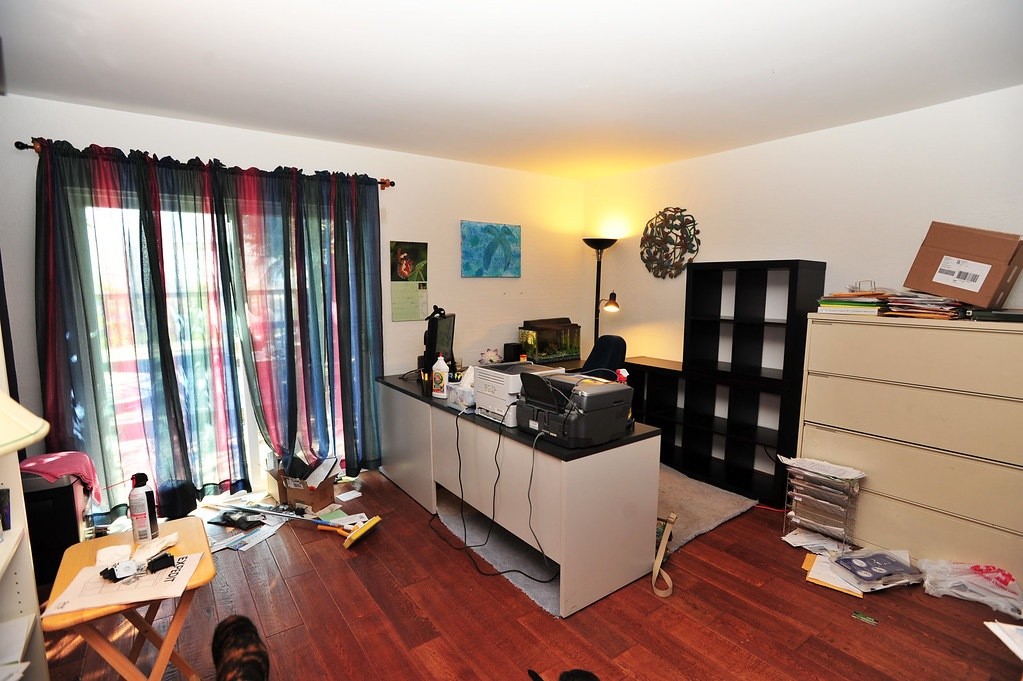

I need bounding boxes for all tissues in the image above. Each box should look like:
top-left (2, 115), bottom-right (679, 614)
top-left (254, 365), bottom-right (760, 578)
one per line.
top-left (445, 365), bottom-right (476, 414)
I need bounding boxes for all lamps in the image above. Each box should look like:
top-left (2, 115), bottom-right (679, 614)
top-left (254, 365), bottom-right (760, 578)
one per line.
top-left (0, 396), bottom-right (50, 459)
top-left (583, 238), bottom-right (618, 344)
top-left (480, 349), bottom-right (502, 363)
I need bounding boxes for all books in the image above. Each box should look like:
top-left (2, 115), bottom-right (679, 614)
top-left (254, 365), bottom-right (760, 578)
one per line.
top-left (817, 290), bottom-right (966, 319)
top-left (828, 554), bottom-right (922, 592)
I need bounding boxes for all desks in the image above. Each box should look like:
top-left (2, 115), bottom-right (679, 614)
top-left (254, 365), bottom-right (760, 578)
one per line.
top-left (40, 516), bottom-right (217, 681)
top-left (375, 359), bottom-right (663, 616)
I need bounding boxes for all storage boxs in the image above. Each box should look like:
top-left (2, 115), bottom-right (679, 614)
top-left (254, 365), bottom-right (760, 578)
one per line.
top-left (901, 221), bottom-right (1023, 311)
top-left (281, 472), bottom-right (339, 513)
top-left (264, 458), bottom-right (289, 504)
top-left (519, 317), bottom-right (581, 363)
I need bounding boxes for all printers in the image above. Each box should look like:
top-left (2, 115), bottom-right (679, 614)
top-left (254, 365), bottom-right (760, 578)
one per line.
top-left (472, 360), bottom-right (633, 450)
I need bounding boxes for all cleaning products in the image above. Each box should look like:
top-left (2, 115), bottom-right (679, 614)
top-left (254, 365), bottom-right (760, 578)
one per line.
top-left (433, 352), bottom-right (449, 398)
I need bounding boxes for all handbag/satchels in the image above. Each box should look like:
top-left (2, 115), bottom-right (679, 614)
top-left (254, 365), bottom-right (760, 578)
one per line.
top-left (651, 513), bottom-right (677, 597)
top-left (916, 557), bottom-right (1023, 619)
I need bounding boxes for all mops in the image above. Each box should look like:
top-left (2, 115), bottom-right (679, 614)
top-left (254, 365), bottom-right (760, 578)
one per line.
top-left (214, 503), bottom-right (381, 550)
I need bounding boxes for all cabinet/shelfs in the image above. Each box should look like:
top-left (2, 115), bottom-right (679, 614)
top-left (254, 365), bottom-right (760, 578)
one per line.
top-left (793, 313), bottom-right (1023, 599)
top-left (0, 327), bottom-right (53, 681)
top-left (624, 355), bottom-right (685, 464)
top-left (782, 466), bottom-right (857, 557)
top-left (684, 259), bottom-right (827, 509)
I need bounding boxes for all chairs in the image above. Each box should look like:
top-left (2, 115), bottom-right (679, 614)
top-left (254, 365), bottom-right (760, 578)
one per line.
top-left (576, 334), bottom-right (627, 382)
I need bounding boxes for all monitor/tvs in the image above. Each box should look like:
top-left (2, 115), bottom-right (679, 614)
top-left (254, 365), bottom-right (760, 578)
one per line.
top-left (422, 313), bottom-right (455, 378)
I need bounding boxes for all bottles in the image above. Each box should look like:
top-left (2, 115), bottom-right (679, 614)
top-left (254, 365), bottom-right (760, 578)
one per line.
top-left (432, 353), bottom-right (449, 399)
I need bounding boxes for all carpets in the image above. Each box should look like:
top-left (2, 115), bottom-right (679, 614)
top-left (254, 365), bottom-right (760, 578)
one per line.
top-left (378, 461), bottom-right (759, 617)
